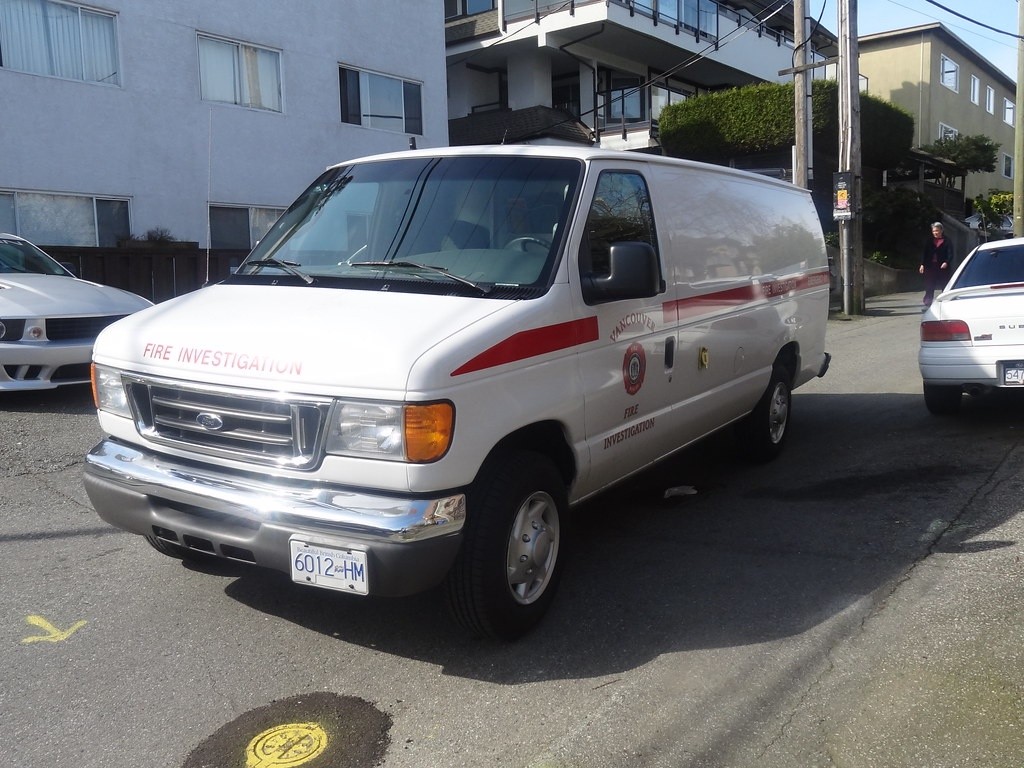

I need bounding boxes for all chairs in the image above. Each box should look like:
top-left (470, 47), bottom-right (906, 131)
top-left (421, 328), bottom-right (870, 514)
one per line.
top-left (427, 222), bottom-right (491, 253)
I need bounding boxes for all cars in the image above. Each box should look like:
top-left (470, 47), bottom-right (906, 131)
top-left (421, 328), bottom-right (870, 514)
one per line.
top-left (917, 238), bottom-right (1024, 418)
top-left (0, 232), bottom-right (156, 401)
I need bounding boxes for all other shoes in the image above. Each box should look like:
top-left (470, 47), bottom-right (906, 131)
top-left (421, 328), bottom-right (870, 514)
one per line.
top-left (921, 305), bottom-right (930, 312)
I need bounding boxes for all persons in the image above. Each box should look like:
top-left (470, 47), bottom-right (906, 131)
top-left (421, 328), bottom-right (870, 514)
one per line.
top-left (919, 222), bottom-right (953, 311)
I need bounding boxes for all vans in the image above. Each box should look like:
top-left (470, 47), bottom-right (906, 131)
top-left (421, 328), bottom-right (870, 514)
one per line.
top-left (81, 144), bottom-right (833, 645)
